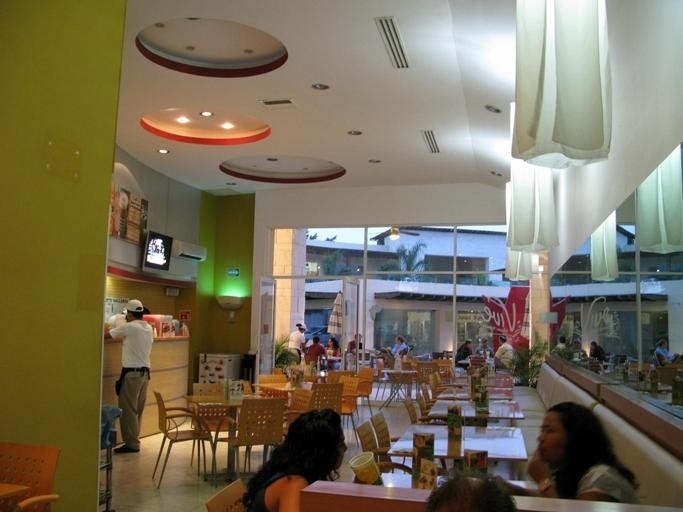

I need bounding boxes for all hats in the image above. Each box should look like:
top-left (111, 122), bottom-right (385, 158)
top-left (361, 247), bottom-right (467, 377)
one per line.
top-left (125, 299), bottom-right (143, 312)
top-left (481, 336), bottom-right (488, 342)
top-left (297, 323), bottom-right (307, 331)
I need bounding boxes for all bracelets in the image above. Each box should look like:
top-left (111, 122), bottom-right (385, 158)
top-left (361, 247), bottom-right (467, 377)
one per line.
top-left (538, 477), bottom-right (554, 492)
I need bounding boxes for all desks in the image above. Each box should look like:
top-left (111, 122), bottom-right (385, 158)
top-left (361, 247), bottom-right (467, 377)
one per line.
top-left (426, 358), bottom-right (528, 426)
top-left (0, 475), bottom-right (31, 504)
top-left (387, 423), bottom-right (528, 473)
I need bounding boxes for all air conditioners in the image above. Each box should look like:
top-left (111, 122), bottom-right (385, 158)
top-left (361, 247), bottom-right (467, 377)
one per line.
top-left (170, 240), bottom-right (208, 263)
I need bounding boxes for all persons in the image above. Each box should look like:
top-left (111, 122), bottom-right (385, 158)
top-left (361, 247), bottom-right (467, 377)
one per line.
top-left (556, 336), bottom-right (608, 373)
top-left (103, 299), bottom-right (156, 453)
top-left (286, 323), bottom-right (364, 373)
top-left (241, 409), bottom-right (371, 512)
top-left (386, 336), bottom-right (407, 361)
top-left (492, 401), bottom-right (645, 506)
top-left (652, 339), bottom-right (671, 369)
top-left (107, 306), bottom-right (127, 329)
top-left (423, 467), bottom-right (519, 511)
top-left (456, 335), bottom-right (514, 373)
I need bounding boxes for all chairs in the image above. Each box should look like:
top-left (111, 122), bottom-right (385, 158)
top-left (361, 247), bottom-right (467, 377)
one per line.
top-left (204, 478), bottom-right (247, 511)
top-left (0, 440), bottom-right (61, 511)
top-left (354, 349), bottom-right (456, 488)
top-left (151, 382), bottom-right (283, 490)
top-left (254, 352), bottom-right (376, 456)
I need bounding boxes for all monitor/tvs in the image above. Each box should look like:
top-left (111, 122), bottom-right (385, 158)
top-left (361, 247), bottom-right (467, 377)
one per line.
top-left (141, 230), bottom-right (174, 276)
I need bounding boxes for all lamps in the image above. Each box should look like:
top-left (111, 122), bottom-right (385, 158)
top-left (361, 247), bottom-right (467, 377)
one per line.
top-left (214, 290), bottom-right (244, 312)
top-left (388, 225), bottom-right (399, 240)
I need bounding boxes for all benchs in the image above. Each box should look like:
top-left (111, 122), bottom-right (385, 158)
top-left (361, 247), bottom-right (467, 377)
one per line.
top-left (509, 353), bottom-right (682, 507)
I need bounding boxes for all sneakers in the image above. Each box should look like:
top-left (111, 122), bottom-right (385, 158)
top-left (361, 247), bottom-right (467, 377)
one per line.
top-left (113, 443), bottom-right (139, 453)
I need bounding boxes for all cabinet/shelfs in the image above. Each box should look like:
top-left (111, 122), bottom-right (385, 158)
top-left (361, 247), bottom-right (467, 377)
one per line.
top-left (98, 402), bottom-right (120, 511)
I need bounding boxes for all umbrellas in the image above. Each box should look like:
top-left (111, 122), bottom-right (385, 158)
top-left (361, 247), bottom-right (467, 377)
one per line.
top-left (328, 289), bottom-right (345, 341)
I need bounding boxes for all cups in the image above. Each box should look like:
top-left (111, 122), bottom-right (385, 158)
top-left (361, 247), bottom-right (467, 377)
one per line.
top-left (349, 451), bottom-right (384, 487)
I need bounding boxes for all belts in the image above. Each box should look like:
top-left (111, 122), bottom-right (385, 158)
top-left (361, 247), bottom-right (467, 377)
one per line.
top-left (121, 367), bottom-right (149, 372)
